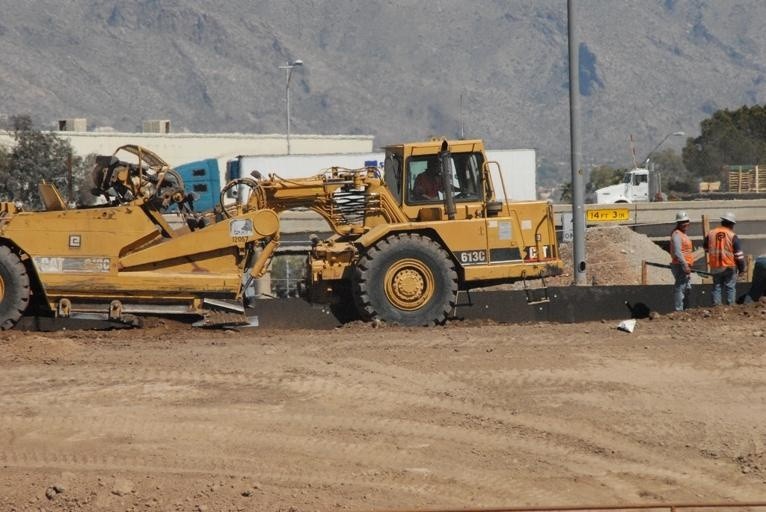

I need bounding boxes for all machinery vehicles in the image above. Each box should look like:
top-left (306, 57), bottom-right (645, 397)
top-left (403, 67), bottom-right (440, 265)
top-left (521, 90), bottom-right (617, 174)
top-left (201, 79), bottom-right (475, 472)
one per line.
top-left (0, 136), bottom-right (566, 333)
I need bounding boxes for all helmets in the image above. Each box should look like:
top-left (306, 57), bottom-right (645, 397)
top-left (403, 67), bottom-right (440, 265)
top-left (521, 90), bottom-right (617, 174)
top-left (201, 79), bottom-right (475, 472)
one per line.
top-left (674, 211), bottom-right (690, 222)
top-left (720, 213), bottom-right (737, 225)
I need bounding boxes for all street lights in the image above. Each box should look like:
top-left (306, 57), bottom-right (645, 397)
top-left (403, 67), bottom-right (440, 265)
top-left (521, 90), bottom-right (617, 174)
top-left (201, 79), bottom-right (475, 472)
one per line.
top-left (639, 130), bottom-right (685, 168)
top-left (284, 58), bottom-right (304, 155)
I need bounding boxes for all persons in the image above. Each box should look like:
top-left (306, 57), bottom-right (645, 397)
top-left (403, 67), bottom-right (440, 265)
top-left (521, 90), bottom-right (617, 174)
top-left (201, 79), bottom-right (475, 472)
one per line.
top-left (413, 159), bottom-right (461, 202)
top-left (703, 211), bottom-right (748, 306)
top-left (669, 210), bottom-right (694, 311)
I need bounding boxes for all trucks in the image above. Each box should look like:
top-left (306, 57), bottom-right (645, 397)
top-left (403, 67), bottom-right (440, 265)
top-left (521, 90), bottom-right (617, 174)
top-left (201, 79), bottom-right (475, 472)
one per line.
top-left (152, 147), bottom-right (538, 248)
top-left (594, 157), bottom-right (658, 204)
